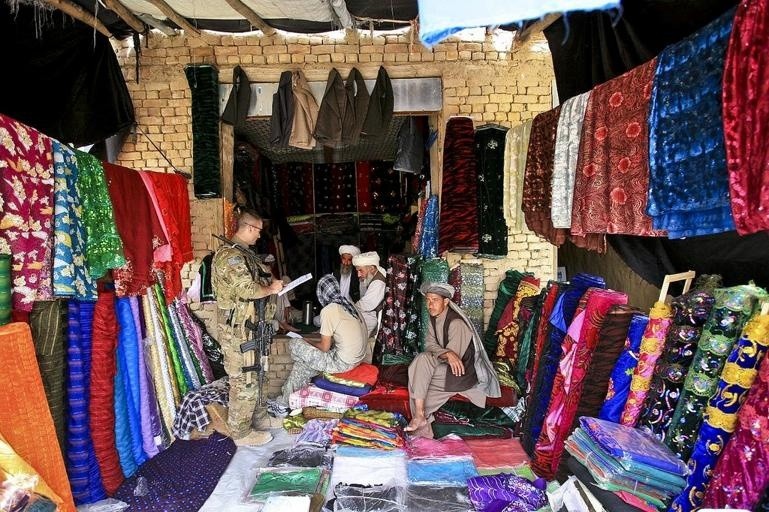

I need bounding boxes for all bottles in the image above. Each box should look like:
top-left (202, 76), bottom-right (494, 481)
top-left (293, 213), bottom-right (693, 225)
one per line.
top-left (302, 301), bottom-right (312, 326)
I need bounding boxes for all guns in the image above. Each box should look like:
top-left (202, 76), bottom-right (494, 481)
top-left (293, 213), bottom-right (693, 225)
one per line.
top-left (240, 273), bottom-right (272, 408)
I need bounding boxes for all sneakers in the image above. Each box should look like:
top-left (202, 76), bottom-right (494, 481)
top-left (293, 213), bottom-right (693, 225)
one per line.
top-left (256, 415), bottom-right (284, 430)
top-left (266, 399), bottom-right (289, 419)
top-left (234, 431), bottom-right (273, 447)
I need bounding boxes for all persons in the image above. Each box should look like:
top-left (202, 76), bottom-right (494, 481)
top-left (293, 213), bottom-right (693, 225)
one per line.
top-left (266, 274), bottom-right (367, 419)
top-left (402, 283), bottom-right (502, 442)
top-left (211, 208), bottom-right (287, 446)
top-left (352, 251), bottom-right (387, 339)
top-left (334, 244), bottom-right (361, 304)
top-left (256, 253), bottom-right (302, 335)
